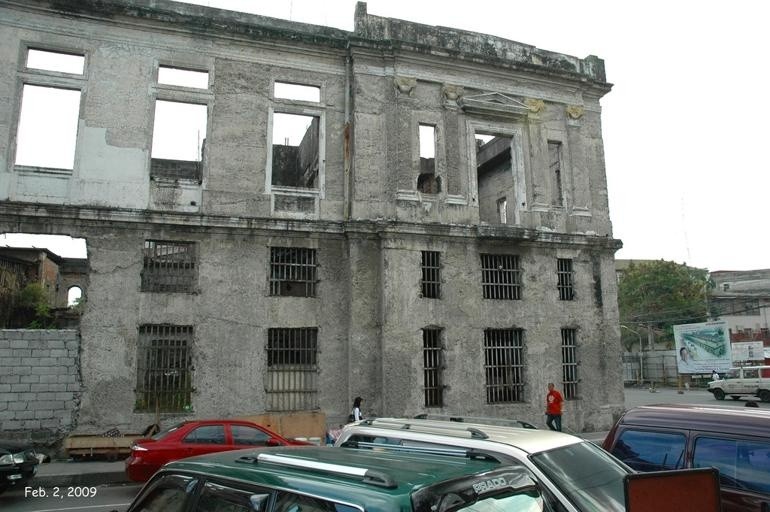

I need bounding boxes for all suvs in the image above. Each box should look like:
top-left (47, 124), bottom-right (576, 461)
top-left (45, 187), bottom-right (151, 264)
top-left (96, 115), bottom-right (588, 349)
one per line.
top-left (706, 366), bottom-right (770, 401)
top-left (334, 416), bottom-right (638, 511)
top-left (127, 447), bottom-right (550, 510)
top-left (604, 404), bottom-right (770, 510)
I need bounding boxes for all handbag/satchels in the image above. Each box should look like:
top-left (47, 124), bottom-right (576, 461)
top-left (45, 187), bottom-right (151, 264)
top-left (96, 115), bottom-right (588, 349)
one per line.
top-left (349, 414), bottom-right (363, 422)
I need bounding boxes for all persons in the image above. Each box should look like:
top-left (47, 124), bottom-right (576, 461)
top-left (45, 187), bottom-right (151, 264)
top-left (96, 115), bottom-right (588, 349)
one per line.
top-left (677, 347), bottom-right (699, 374)
top-left (712, 370), bottom-right (719, 381)
top-left (544, 383), bottom-right (564, 432)
top-left (350, 397), bottom-right (365, 424)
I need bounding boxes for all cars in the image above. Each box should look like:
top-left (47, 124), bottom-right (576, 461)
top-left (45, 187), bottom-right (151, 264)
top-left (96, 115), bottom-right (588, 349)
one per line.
top-left (126, 417), bottom-right (316, 481)
top-left (0, 438), bottom-right (36, 493)
